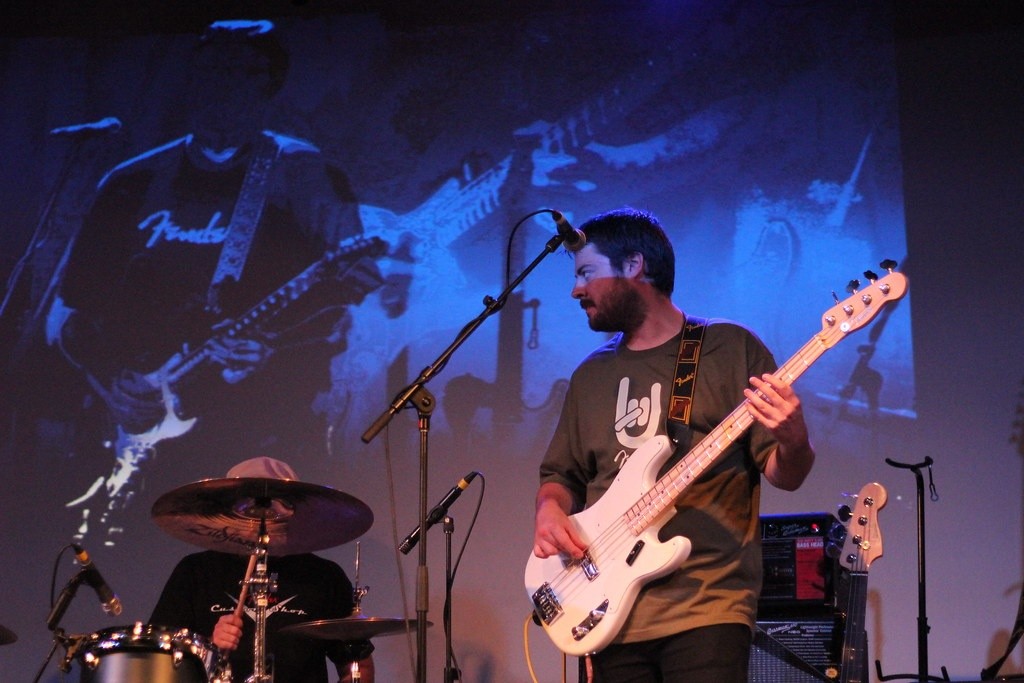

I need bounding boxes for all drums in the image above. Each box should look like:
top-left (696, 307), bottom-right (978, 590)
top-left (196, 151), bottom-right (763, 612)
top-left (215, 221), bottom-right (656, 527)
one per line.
top-left (77, 621), bottom-right (231, 683)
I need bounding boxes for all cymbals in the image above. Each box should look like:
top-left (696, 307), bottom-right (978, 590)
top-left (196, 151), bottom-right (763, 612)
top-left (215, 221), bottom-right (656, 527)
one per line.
top-left (275, 608), bottom-right (436, 645)
top-left (151, 475), bottom-right (375, 556)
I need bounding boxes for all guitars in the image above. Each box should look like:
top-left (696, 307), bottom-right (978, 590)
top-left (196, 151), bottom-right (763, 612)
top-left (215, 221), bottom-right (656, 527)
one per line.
top-left (67, 233), bottom-right (387, 510)
top-left (521, 259), bottom-right (909, 657)
top-left (823, 482), bottom-right (889, 683)
top-left (350, 50), bottom-right (677, 369)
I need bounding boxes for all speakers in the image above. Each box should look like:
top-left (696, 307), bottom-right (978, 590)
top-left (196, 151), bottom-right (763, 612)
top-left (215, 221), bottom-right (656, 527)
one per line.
top-left (745, 619), bottom-right (869, 683)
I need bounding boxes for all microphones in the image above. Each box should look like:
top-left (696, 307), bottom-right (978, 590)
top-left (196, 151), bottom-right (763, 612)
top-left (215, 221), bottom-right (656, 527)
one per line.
top-left (397, 469), bottom-right (478, 556)
top-left (51, 117), bottom-right (124, 138)
top-left (72, 544), bottom-right (122, 618)
top-left (552, 210), bottom-right (586, 251)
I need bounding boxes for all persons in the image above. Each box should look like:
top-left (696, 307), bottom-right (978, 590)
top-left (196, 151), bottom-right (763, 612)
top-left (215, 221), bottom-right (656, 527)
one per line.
top-left (46, 17), bottom-right (384, 626)
top-left (531, 208), bottom-right (817, 683)
top-left (144, 456), bottom-right (378, 683)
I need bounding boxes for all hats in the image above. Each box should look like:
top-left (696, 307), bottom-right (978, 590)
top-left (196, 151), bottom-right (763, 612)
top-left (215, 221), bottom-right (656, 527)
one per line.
top-left (226, 457), bottom-right (299, 481)
